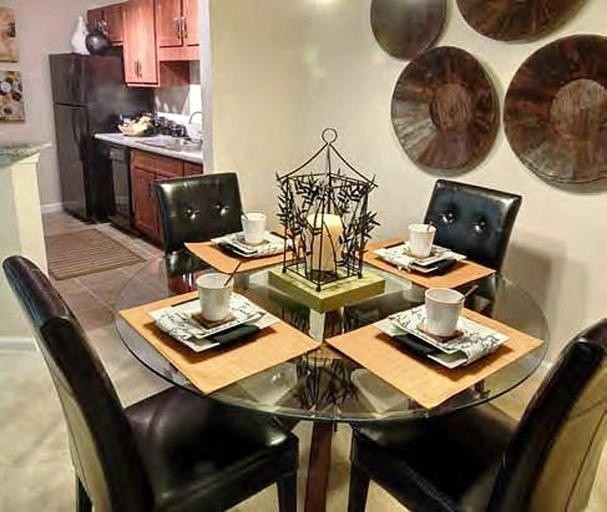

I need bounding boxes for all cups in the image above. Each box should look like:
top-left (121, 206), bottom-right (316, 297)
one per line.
top-left (242, 213), bottom-right (268, 245)
top-left (195, 271), bottom-right (236, 323)
top-left (404, 223), bottom-right (438, 255)
top-left (422, 287), bottom-right (468, 338)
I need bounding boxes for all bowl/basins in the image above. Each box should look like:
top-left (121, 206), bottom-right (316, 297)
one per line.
top-left (117, 124), bottom-right (150, 136)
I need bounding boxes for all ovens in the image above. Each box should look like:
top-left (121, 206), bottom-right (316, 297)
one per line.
top-left (92, 139), bottom-right (132, 233)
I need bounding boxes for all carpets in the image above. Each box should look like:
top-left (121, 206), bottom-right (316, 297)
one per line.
top-left (44, 228), bottom-right (145, 281)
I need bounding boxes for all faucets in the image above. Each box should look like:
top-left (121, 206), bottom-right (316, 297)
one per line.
top-left (188, 110), bottom-right (204, 149)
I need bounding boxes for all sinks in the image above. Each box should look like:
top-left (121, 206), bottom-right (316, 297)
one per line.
top-left (135, 133), bottom-right (203, 152)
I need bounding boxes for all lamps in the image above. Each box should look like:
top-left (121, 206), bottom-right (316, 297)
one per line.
top-left (274, 127), bottom-right (386, 313)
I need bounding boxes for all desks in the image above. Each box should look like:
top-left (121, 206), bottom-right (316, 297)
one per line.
top-left (112, 239), bottom-right (549, 511)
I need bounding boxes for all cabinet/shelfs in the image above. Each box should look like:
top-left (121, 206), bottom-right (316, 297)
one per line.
top-left (130, 147), bottom-right (204, 252)
top-left (87, 4), bottom-right (123, 46)
top-left (151, 1), bottom-right (198, 62)
top-left (120, 0), bottom-right (189, 87)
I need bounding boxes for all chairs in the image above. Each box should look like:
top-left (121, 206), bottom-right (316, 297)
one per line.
top-left (153, 173), bottom-right (247, 290)
top-left (350, 319), bottom-right (607, 512)
top-left (1, 254), bottom-right (302, 512)
top-left (419, 178), bottom-right (521, 395)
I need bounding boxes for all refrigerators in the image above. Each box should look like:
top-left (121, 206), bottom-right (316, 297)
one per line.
top-left (48, 52), bottom-right (158, 226)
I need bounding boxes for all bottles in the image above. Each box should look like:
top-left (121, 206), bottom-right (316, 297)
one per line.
top-left (85, 28), bottom-right (112, 55)
top-left (141, 110), bottom-right (185, 138)
top-left (71, 16), bottom-right (92, 55)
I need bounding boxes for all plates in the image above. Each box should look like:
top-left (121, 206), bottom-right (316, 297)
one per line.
top-left (504, 32), bottom-right (607, 185)
top-left (209, 229), bottom-right (291, 259)
top-left (456, 2), bottom-right (591, 44)
top-left (369, 303), bottom-right (513, 370)
top-left (392, 46), bottom-right (498, 173)
top-left (371, 241), bottom-right (468, 275)
top-left (371, 0), bottom-right (448, 62)
top-left (142, 294), bottom-right (281, 353)
